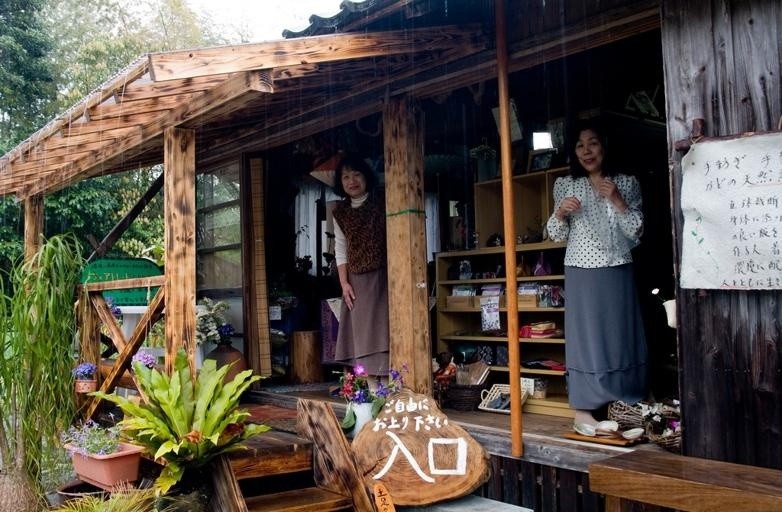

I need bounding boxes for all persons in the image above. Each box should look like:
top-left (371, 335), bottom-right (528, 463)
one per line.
top-left (545, 121), bottom-right (648, 437)
top-left (331, 155), bottom-right (392, 399)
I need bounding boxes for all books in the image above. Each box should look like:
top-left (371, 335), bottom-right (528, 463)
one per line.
top-left (445, 293), bottom-right (541, 308)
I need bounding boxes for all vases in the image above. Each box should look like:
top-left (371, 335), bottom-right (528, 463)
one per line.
top-left (71, 362), bottom-right (97, 379)
top-left (57, 415), bottom-right (123, 460)
top-left (194, 295), bottom-right (237, 346)
top-left (132, 348), bottom-right (155, 371)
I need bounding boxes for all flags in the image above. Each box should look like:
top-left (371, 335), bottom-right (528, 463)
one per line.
top-left (75, 379), bottom-right (97, 393)
top-left (64, 440), bottom-right (145, 492)
top-left (207, 339), bottom-right (248, 385)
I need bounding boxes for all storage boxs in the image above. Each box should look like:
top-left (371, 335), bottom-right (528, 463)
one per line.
top-left (587, 450), bottom-right (782, 511)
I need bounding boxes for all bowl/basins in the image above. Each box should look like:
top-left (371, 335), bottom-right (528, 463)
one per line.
top-left (587, 175), bottom-right (603, 193)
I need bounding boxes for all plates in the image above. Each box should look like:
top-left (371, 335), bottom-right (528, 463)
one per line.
top-left (436, 165), bottom-right (574, 419)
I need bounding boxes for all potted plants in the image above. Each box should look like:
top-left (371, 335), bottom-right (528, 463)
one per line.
top-left (527, 148), bottom-right (558, 173)
